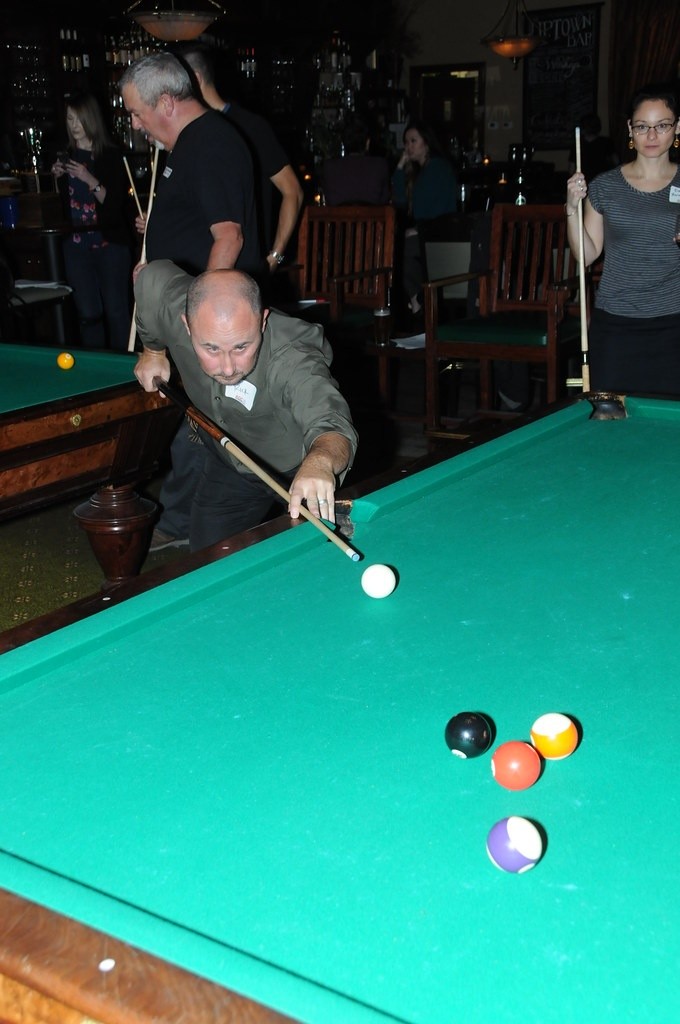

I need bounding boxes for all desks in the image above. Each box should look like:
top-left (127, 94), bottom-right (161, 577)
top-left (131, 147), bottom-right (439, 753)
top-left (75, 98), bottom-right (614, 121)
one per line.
top-left (0, 345), bottom-right (174, 580)
top-left (0, 388), bottom-right (680, 1024)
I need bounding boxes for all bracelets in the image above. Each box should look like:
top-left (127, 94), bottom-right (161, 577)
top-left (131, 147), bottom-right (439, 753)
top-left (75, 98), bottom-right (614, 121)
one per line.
top-left (563, 203), bottom-right (577, 217)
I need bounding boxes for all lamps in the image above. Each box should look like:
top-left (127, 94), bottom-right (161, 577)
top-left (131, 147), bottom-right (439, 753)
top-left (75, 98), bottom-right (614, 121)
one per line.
top-left (481, 0), bottom-right (542, 70)
top-left (124, 0), bottom-right (224, 40)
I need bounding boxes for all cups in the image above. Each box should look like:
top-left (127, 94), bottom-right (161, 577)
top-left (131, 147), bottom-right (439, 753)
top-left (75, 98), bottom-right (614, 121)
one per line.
top-left (0, 195), bottom-right (18, 229)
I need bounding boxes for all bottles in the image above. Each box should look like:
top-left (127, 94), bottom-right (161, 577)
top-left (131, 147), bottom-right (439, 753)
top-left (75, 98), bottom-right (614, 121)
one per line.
top-left (498, 172), bottom-right (507, 185)
top-left (515, 191), bottom-right (526, 206)
top-left (514, 169), bottom-right (526, 185)
top-left (0, 1), bottom-right (493, 171)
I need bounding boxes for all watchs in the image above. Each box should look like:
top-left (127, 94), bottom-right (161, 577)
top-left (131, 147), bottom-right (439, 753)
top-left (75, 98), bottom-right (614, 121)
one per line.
top-left (269, 251), bottom-right (286, 264)
top-left (91, 183), bottom-right (102, 192)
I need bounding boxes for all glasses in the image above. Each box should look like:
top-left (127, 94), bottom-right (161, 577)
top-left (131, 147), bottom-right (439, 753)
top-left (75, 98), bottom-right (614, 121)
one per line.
top-left (630, 117), bottom-right (678, 136)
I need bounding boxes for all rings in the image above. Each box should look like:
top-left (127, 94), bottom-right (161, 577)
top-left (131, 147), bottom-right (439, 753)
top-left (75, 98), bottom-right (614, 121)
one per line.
top-left (576, 179), bottom-right (582, 186)
top-left (579, 186), bottom-right (584, 191)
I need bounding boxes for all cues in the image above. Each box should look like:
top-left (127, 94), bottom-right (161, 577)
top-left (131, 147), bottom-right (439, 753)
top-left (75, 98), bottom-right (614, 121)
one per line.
top-left (574, 128), bottom-right (592, 392)
top-left (122, 156), bottom-right (144, 221)
top-left (152, 376), bottom-right (360, 562)
top-left (128, 146), bottom-right (162, 353)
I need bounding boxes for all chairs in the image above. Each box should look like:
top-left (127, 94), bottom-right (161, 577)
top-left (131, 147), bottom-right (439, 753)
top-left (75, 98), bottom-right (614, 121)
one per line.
top-left (426, 202), bottom-right (575, 447)
top-left (294, 206), bottom-right (395, 415)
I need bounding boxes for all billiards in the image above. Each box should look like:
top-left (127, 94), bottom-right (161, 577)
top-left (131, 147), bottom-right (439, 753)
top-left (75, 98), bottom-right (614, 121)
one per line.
top-left (57, 352), bottom-right (75, 370)
top-left (530, 711), bottom-right (577, 760)
top-left (485, 815), bottom-right (544, 873)
top-left (490, 741), bottom-right (541, 790)
top-left (360, 564), bottom-right (396, 599)
top-left (445, 711), bottom-right (491, 758)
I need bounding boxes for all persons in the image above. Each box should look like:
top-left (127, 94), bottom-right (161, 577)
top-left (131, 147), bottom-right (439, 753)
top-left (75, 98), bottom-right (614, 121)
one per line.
top-left (314, 124), bottom-right (391, 238)
top-left (130, 257), bottom-right (360, 553)
top-left (564, 86), bottom-right (680, 398)
top-left (49, 97), bottom-right (137, 355)
top-left (386, 123), bottom-right (460, 319)
top-left (567, 114), bottom-right (610, 175)
top-left (114, 49), bottom-right (304, 312)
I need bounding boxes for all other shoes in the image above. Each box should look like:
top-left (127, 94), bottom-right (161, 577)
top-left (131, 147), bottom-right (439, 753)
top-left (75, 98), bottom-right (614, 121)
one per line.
top-left (149, 529), bottom-right (190, 551)
